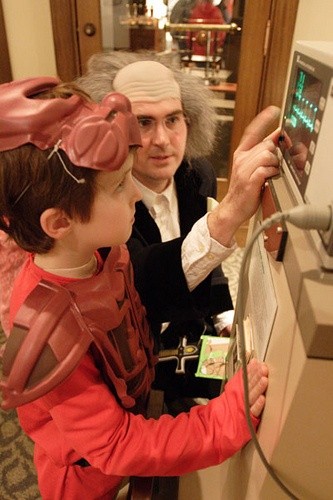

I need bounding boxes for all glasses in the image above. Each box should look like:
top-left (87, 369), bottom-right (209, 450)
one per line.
top-left (138, 115), bottom-right (187, 133)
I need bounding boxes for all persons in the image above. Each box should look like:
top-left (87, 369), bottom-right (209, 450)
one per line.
top-left (0, 77), bottom-right (268, 500)
top-left (74, 49), bottom-right (282, 395)
top-left (168, 0), bottom-right (239, 57)
top-left (282, 129), bottom-right (308, 171)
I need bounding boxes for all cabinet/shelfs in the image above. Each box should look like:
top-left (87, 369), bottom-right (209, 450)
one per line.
top-left (220, 150), bottom-right (333, 500)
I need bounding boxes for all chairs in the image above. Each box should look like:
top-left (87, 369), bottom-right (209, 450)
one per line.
top-left (163, 23), bottom-right (242, 86)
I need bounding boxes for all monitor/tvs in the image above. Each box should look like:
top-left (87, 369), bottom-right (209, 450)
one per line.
top-left (278, 67), bottom-right (323, 186)
